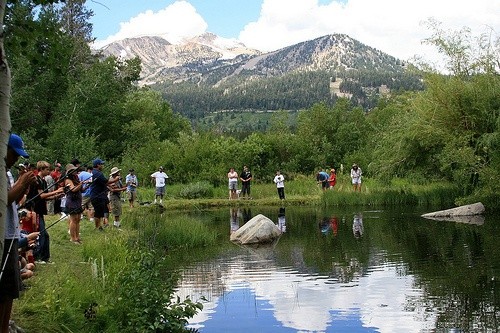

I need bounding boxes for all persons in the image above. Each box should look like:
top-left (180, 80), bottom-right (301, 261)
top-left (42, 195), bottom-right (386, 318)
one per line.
top-left (107, 166), bottom-right (130, 227)
top-left (240, 164), bottom-right (252, 197)
top-left (19, 160), bottom-right (94, 215)
top-left (24, 160), bottom-right (65, 265)
top-left (227, 167), bottom-right (239, 199)
top-left (90, 159), bottom-right (121, 230)
top-left (0, 131), bottom-right (35, 333)
top-left (65, 164), bottom-right (92, 244)
top-left (125, 168), bottom-right (138, 208)
top-left (326, 169), bottom-right (337, 191)
top-left (316, 171), bottom-right (330, 191)
top-left (351, 163), bottom-right (362, 193)
top-left (17, 208), bottom-right (41, 280)
top-left (150, 166), bottom-right (169, 204)
top-left (272, 170), bottom-right (285, 201)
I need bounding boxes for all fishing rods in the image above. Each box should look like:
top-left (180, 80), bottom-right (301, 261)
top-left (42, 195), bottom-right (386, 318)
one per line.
top-left (39, 190), bottom-right (110, 234)
top-left (17, 147), bottom-right (109, 210)
top-left (121, 163), bottom-right (140, 179)
top-left (63, 155), bottom-right (127, 195)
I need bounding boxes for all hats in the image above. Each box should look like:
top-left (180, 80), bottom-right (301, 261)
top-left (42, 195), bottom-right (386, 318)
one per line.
top-left (93, 159), bottom-right (106, 165)
top-left (65, 165), bottom-right (78, 175)
top-left (109, 167), bottom-right (121, 175)
top-left (8, 134), bottom-right (29, 159)
top-left (70, 158), bottom-right (81, 165)
top-left (129, 168), bottom-right (134, 171)
top-left (159, 166), bottom-right (163, 170)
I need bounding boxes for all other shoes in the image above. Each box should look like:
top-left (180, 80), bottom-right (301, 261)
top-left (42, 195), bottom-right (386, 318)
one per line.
top-left (99, 226), bottom-right (104, 232)
top-left (34, 259), bottom-right (46, 264)
top-left (23, 285), bottom-right (30, 289)
top-left (70, 238), bottom-right (82, 245)
